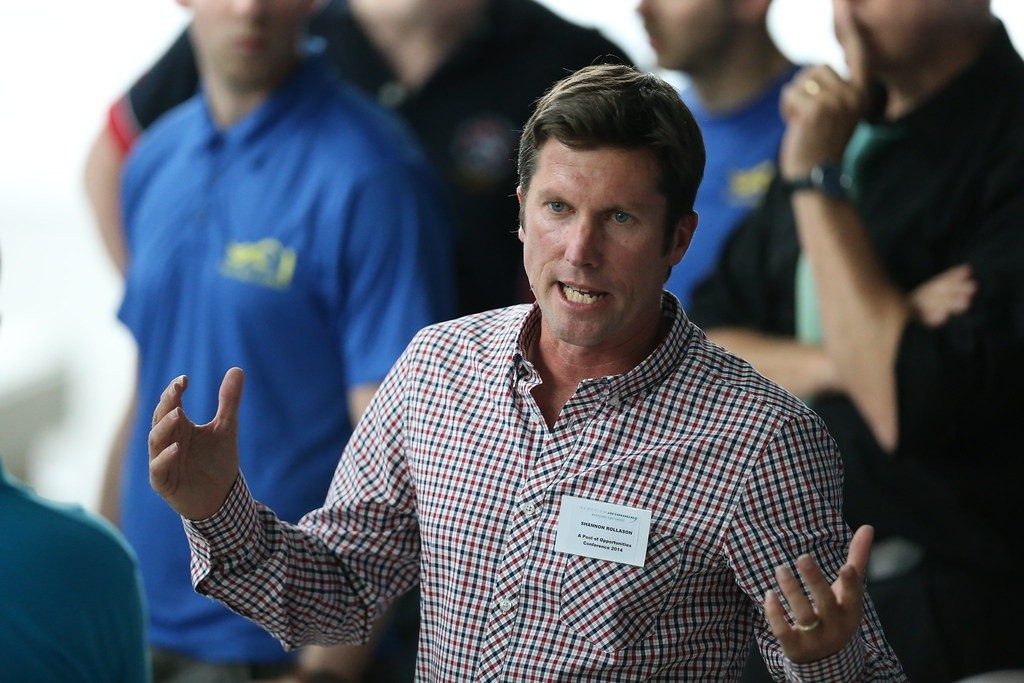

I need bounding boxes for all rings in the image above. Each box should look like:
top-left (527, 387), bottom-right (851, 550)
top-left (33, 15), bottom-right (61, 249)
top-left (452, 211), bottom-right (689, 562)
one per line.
top-left (795, 615), bottom-right (820, 632)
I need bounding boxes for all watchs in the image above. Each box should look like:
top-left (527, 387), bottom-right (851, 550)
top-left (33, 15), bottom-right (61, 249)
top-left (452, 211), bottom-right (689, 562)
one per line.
top-left (780, 161), bottom-right (845, 200)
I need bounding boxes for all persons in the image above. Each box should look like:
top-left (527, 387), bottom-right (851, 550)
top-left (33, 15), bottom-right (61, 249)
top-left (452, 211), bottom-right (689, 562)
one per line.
top-left (148, 62), bottom-right (908, 683)
top-left (0, 0), bottom-right (1024, 683)
